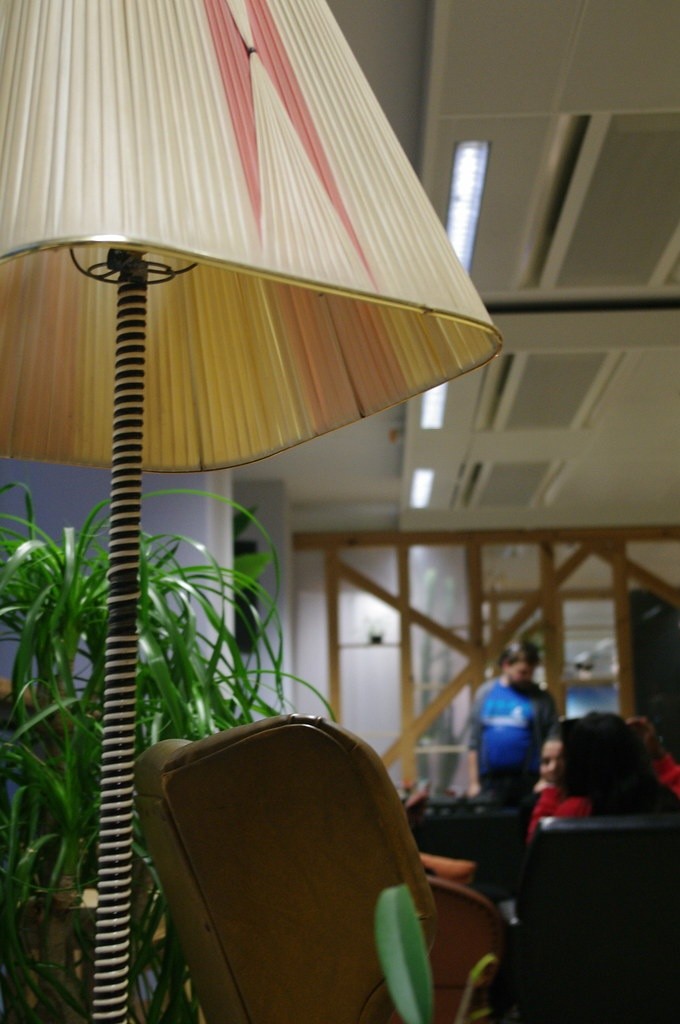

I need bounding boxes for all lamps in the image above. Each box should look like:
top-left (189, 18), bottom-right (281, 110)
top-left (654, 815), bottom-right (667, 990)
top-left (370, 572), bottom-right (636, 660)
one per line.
top-left (0, 1), bottom-right (502, 1024)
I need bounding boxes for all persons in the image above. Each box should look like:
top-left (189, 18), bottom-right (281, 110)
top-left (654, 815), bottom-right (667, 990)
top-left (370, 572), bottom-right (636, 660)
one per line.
top-left (465, 639), bottom-right (559, 803)
top-left (525, 709), bottom-right (680, 850)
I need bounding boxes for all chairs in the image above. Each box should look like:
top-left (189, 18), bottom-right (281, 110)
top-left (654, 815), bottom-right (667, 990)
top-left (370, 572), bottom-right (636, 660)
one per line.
top-left (133, 714), bottom-right (680, 1024)
